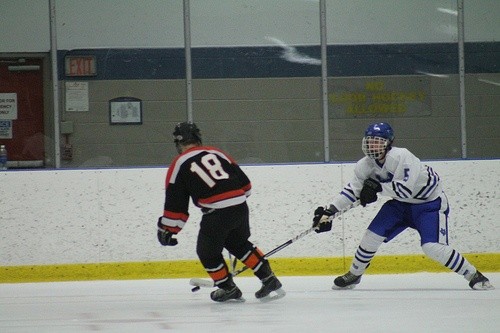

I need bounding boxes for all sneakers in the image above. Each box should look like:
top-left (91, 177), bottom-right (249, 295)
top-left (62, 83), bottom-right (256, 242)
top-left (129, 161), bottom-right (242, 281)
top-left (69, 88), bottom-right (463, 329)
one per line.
top-left (330, 271), bottom-right (362, 291)
top-left (254, 262), bottom-right (285, 303)
top-left (210, 275), bottom-right (242, 303)
top-left (468, 270), bottom-right (492, 291)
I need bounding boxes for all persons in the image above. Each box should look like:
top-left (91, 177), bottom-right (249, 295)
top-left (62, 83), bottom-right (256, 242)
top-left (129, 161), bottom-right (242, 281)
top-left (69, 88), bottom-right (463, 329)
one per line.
top-left (156, 121), bottom-right (282, 302)
top-left (313, 121), bottom-right (489, 288)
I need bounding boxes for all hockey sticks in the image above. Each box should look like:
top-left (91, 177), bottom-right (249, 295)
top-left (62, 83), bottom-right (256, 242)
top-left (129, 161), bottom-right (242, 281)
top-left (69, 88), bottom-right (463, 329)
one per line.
top-left (227, 250), bottom-right (238, 272)
top-left (190, 200), bottom-right (361, 287)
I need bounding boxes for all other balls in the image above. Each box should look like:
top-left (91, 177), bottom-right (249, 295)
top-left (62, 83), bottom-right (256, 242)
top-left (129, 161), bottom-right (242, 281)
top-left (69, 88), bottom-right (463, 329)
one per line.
top-left (191, 286), bottom-right (200, 292)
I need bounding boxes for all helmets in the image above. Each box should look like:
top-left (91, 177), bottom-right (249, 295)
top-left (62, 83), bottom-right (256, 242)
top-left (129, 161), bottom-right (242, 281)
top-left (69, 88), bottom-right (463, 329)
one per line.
top-left (173, 122), bottom-right (202, 143)
top-left (365, 122), bottom-right (395, 141)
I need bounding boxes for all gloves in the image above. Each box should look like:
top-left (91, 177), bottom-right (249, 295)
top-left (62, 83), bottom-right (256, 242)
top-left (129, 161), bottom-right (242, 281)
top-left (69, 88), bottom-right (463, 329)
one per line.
top-left (156, 228), bottom-right (177, 247)
top-left (360, 176), bottom-right (383, 208)
top-left (311, 206), bottom-right (334, 233)
